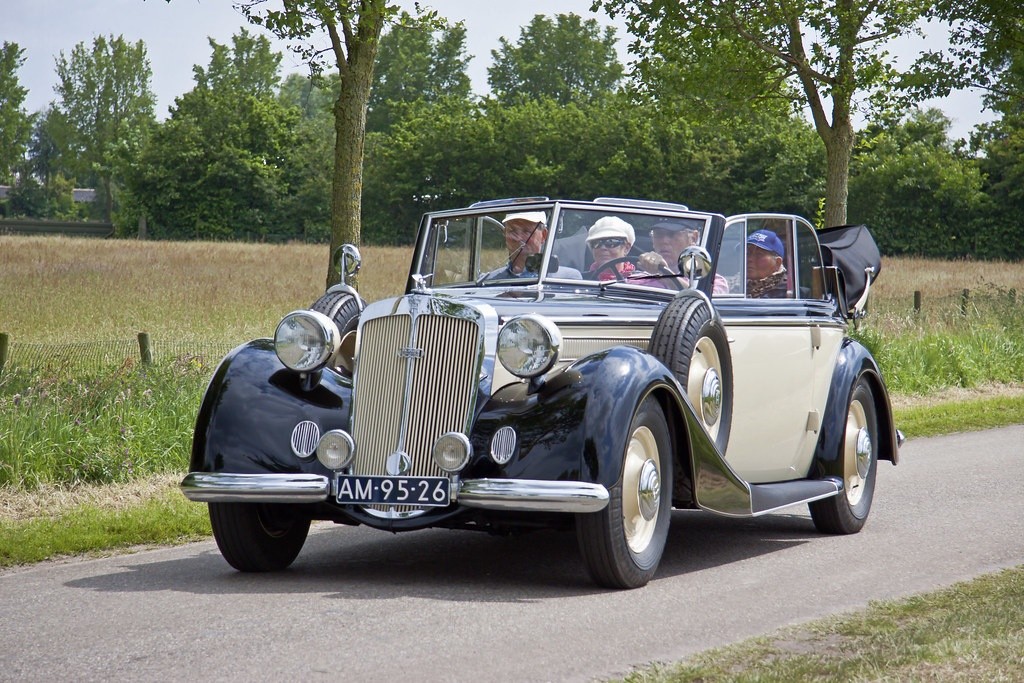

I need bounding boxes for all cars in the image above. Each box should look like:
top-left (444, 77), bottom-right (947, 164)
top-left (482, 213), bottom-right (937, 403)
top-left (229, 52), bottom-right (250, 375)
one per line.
top-left (177, 194), bottom-right (909, 588)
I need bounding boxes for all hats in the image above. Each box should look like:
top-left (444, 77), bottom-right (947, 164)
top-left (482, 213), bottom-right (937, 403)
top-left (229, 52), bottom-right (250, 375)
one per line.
top-left (584, 215), bottom-right (636, 247)
top-left (649, 213), bottom-right (704, 232)
top-left (735, 227), bottom-right (785, 259)
top-left (501, 209), bottom-right (547, 226)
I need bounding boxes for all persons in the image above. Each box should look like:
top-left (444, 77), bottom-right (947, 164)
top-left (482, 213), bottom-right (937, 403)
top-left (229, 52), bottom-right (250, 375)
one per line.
top-left (622, 216), bottom-right (729, 298)
top-left (584, 214), bottom-right (636, 286)
top-left (723, 229), bottom-right (805, 299)
top-left (478, 210), bottom-right (583, 280)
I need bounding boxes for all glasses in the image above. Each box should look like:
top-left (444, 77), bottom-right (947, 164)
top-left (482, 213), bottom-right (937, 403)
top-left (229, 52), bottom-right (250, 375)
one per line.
top-left (590, 236), bottom-right (628, 248)
